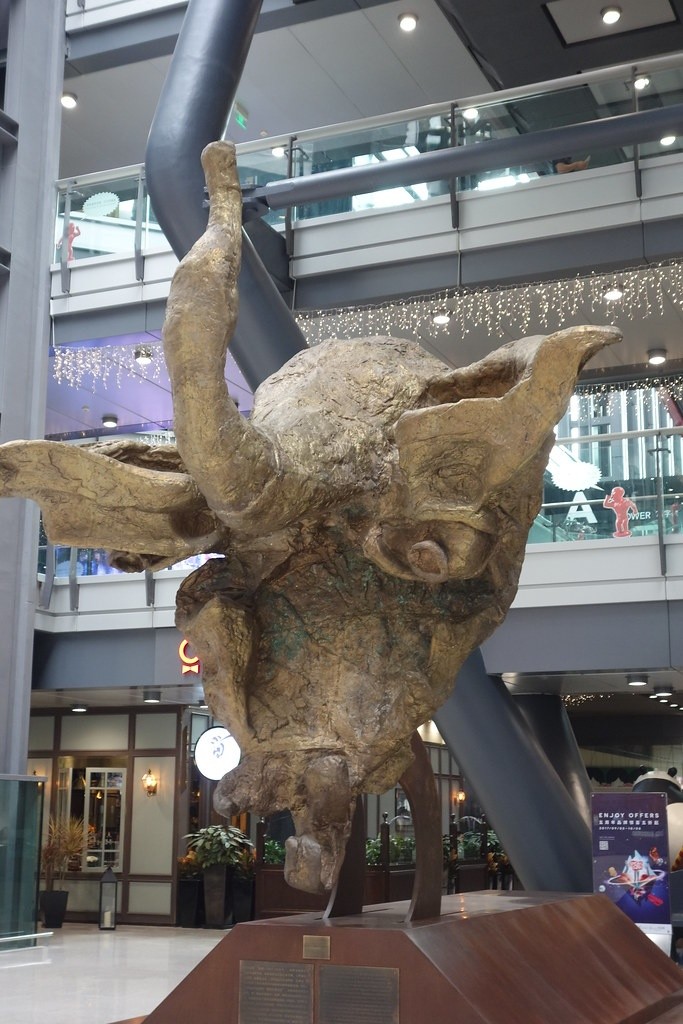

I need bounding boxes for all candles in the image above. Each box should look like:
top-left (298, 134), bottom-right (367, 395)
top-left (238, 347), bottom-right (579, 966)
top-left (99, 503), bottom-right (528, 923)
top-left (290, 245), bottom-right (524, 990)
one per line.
top-left (104, 911), bottom-right (111, 928)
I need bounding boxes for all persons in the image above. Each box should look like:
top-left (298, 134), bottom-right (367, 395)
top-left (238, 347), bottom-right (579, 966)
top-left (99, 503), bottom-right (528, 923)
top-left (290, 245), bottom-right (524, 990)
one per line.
top-left (603, 486), bottom-right (638, 537)
top-left (56, 222), bottom-right (81, 261)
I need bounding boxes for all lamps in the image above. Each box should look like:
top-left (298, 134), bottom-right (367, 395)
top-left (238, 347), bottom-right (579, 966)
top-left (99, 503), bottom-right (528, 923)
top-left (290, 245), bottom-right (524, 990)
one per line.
top-left (430, 309), bottom-right (452, 323)
top-left (626, 675), bottom-right (649, 686)
top-left (602, 284), bottom-right (667, 364)
top-left (199, 701), bottom-right (208, 708)
top-left (143, 691), bottom-right (161, 703)
top-left (397, 13), bottom-right (419, 31)
top-left (649, 687), bottom-right (673, 698)
top-left (142, 769), bottom-right (157, 797)
top-left (600, 6), bottom-right (622, 24)
top-left (72, 704), bottom-right (87, 712)
top-left (135, 350), bottom-right (152, 365)
top-left (102, 417), bottom-right (117, 428)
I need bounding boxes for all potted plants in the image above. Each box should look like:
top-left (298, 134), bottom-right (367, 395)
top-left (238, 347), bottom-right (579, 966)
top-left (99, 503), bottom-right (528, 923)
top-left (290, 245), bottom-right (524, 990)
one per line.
top-left (183, 824), bottom-right (253, 928)
top-left (38, 815), bottom-right (96, 928)
